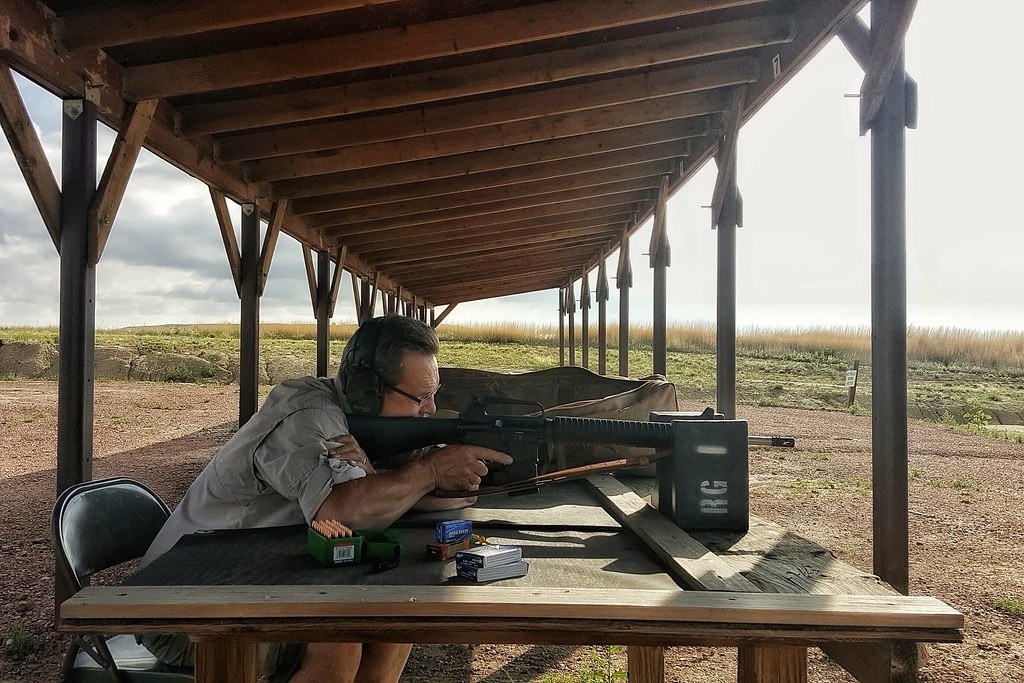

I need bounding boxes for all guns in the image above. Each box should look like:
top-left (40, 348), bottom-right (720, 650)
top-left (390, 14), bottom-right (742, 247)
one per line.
top-left (347, 395), bottom-right (795, 497)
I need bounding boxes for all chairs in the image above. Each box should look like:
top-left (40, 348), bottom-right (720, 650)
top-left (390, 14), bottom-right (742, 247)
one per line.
top-left (46, 480), bottom-right (195, 683)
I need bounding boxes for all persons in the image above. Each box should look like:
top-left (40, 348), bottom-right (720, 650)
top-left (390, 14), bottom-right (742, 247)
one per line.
top-left (127, 317), bottom-right (514, 683)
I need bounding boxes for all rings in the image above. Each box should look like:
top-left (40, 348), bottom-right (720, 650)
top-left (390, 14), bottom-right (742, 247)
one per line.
top-left (363, 458), bottom-right (367, 465)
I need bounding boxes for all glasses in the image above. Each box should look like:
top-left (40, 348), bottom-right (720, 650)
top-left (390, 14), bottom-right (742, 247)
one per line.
top-left (382, 381), bottom-right (441, 408)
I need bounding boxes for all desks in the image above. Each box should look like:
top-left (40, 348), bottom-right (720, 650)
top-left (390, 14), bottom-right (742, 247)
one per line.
top-left (60, 476), bottom-right (965, 683)
